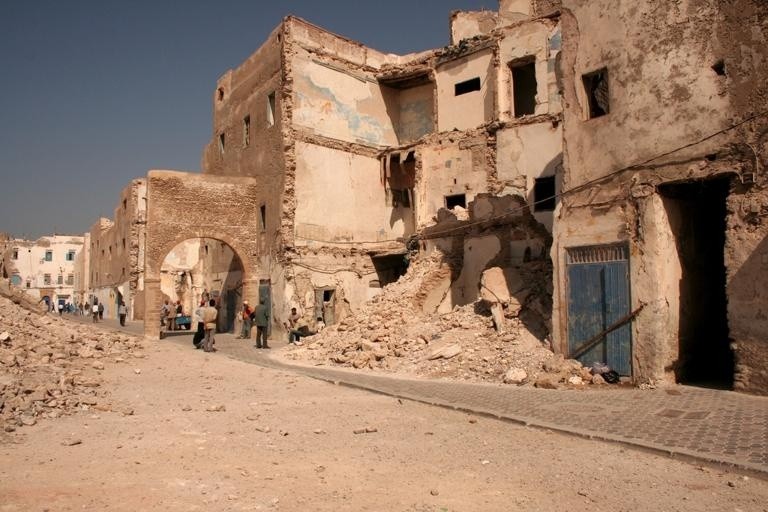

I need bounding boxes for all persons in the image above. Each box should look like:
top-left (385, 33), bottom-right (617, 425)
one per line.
top-left (288, 315), bottom-right (310, 343)
top-left (287, 306), bottom-right (300, 328)
top-left (160, 300), bottom-right (170, 327)
top-left (253, 296), bottom-right (271, 349)
top-left (192, 299), bottom-right (208, 347)
top-left (201, 299), bottom-right (218, 352)
top-left (236, 299), bottom-right (255, 339)
top-left (118, 301), bottom-right (128, 327)
top-left (50, 300), bottom-right (104, 323)
top-left (174, 300), bottom-right (181, 318)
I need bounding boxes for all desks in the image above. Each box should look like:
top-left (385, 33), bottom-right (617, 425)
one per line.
top-left (175, 316), bottom-right (191, 329)
